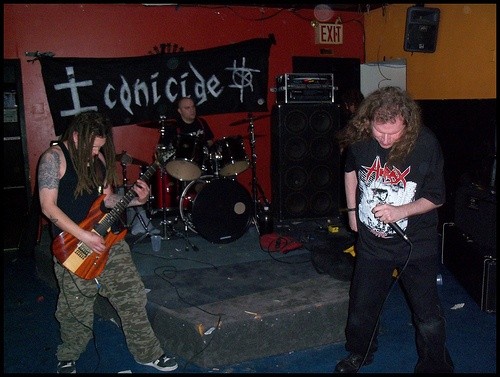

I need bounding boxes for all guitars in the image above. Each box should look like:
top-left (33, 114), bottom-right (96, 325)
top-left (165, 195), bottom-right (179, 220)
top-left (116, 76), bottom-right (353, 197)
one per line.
top-left (50, 154), bottom-right (166, 280)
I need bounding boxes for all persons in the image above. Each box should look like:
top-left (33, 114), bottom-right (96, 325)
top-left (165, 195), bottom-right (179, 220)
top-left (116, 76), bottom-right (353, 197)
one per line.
top-left (38, 110), bottom-right (178, 374)
top-left (335, 86), bottom-right (455, 377)
top-left (158, 98), bottom-right (215, 218)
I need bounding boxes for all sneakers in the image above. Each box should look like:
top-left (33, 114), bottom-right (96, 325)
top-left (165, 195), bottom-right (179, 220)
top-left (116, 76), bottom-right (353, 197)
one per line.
top-left (57, 360), bottom-right (77, 373)
top-left (335, 351), bottom-right (374, 373)
top-left (137, 353), bottom-right (178, 371)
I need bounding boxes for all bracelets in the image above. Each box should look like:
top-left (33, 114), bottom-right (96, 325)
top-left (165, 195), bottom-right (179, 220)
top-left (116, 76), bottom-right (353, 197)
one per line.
top-left (347, 208), bottom-right (356, 211)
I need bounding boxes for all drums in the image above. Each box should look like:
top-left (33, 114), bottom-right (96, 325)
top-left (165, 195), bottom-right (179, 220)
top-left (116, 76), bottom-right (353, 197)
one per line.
top-left (164, 138), bottom-right (211, 181)
top-left (179, 174), bottom-right (255, 245)
top-left (150, 165), bottom-right (183, 216)
top-left (208, 135), bottom-right (250, 177)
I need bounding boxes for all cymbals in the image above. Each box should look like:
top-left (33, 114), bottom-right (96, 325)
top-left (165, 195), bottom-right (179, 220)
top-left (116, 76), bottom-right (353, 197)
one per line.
top-left (136, 120), bottom-right (185, 129)
top-left (116, 150), bottom-right (150, 166)
top-left (228, 113), bottom-right (271, 127)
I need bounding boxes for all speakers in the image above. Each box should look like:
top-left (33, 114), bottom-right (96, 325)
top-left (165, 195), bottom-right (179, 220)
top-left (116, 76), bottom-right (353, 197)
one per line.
top-left (403, 6), bottom-right (440, 53)
top-left (271, 104), bottom-right (343, 223)
top-left (3, 57), bottom-right (37, 251)
top-left (441, 182), bottom-right (497, 314)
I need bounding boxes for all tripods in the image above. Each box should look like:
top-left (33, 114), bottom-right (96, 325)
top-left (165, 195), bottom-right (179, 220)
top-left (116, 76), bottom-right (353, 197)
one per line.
top-left (121, 122), bottom-right (200, 252)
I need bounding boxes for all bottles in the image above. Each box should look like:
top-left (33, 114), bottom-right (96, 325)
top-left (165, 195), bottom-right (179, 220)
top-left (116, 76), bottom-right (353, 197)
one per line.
top-left (260, 206), bottom-right (273, 235)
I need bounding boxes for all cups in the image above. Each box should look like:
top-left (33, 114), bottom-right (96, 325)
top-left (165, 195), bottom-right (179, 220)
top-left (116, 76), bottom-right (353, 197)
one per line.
top-left (151, 235), bottom-right (161, 251)
top-left (437, 274), bottom-right (442, 285)
